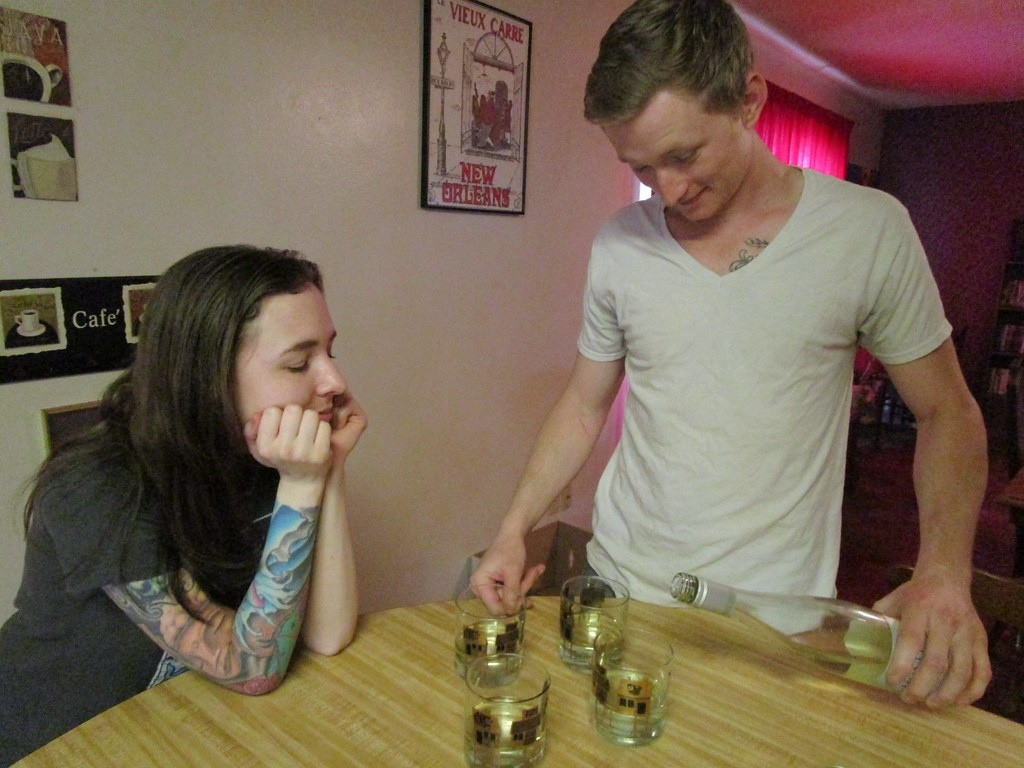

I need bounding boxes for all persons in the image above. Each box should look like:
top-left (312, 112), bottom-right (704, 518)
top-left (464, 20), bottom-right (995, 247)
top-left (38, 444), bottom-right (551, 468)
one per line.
top-left (0, 244), bottom-right (370, 768)
top-left (467, 0), bottom-right (993, 713)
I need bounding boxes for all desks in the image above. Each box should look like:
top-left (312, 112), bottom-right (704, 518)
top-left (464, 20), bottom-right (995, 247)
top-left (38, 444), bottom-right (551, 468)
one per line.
top-left (0, 597), bottom-right (1024, 768)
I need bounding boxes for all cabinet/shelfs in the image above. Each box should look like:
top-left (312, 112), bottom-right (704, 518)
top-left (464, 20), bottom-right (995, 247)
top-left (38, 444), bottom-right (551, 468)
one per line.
top-left (980, 216), bottom-right (1024, 403)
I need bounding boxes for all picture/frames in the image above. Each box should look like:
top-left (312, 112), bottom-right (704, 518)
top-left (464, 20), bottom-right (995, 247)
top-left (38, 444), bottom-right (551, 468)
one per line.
top-left (421, 1), bottom-right (534, 215)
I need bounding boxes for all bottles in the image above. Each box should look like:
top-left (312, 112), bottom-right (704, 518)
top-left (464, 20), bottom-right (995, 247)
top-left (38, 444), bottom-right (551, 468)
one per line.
top-left (669, 572), bottom-right (926, 693)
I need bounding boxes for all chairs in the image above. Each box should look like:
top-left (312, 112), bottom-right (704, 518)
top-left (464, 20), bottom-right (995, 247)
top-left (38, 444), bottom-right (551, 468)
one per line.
top-left (1004, 366), bottom-right (1024, 579)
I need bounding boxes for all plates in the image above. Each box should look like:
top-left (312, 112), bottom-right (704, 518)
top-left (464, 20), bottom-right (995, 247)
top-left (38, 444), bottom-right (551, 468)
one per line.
top-left (16, 324), bottom-right (46, 337)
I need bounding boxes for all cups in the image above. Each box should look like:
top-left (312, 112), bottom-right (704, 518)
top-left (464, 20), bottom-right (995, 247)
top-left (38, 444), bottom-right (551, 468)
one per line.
top-left (464, 653), bottom-right (552, 768)
top-left (452, 584), bottom-right (527, 688)
top-left (15, 309), bottom-right (39, 332)
top-left (1, 53), bottom-right (62, 104)
top-left (11, 153), bottom-right (76, 200)
top-left (559, 574), bottom-right (630, 675)
top-left (589, 626), bottom-right (675, 746)
